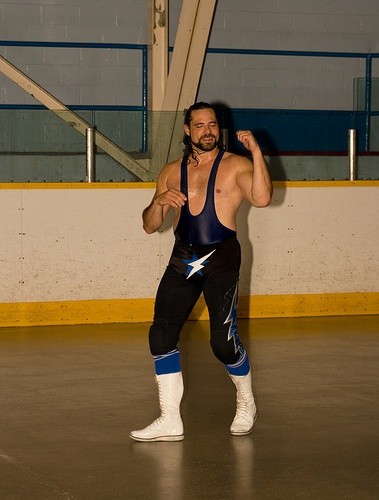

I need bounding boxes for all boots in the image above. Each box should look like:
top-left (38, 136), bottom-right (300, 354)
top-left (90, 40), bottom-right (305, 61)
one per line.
top-left (129, 372), bottom-right (185, 441)
top-left (227, 366), bottom-right (258, 435)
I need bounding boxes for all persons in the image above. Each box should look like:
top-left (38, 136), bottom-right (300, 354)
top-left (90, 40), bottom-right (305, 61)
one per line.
top-left (130, 101), bottom-right (273, 443)
